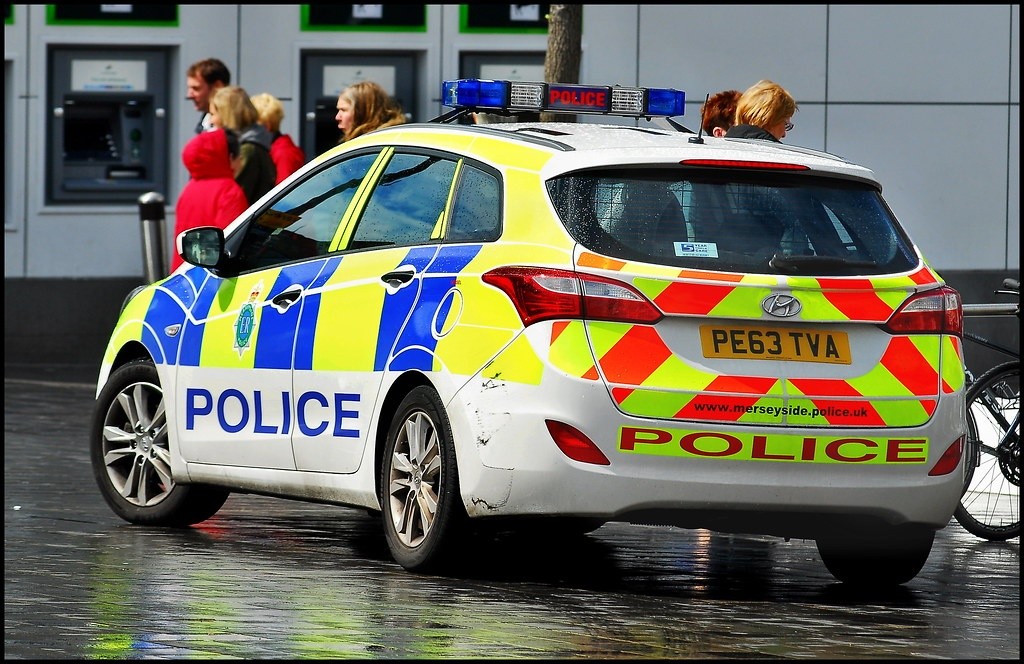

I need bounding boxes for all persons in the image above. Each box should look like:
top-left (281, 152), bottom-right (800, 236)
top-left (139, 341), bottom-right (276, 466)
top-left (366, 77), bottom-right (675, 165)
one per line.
top-left (209, 86), bottom-right (277, 207)
top-left (335, 81), bottom-right (402, 142)
top-left (170, 129), bottom-right (249, 271)
top-left (250, 94), bottom-right (305, 185)
top-left (187, 59), bottom-right (230, 134)
top-left (688, 91), bottom-right (742, 243)
top-left (724, 80), bottom-right (847, 256)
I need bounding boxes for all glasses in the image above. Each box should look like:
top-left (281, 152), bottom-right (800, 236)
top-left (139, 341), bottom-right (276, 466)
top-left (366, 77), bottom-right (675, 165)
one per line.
top-left (783, 118), bottom-right (794, 131)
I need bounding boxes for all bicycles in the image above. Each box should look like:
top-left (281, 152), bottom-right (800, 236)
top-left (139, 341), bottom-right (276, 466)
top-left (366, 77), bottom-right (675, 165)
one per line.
top-left (952, 277), bottom-right (1023, 543)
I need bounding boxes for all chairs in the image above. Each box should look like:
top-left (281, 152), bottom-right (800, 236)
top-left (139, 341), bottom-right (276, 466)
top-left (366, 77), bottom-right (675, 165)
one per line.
top-left (718, 214), bottom-right (788, 254)
top-left (612, 190), bottom-right (689, 259)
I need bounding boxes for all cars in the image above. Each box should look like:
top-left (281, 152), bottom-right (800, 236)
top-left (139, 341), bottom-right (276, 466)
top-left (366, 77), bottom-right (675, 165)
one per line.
top-left (88, 120), bottom-right (973, 599)
